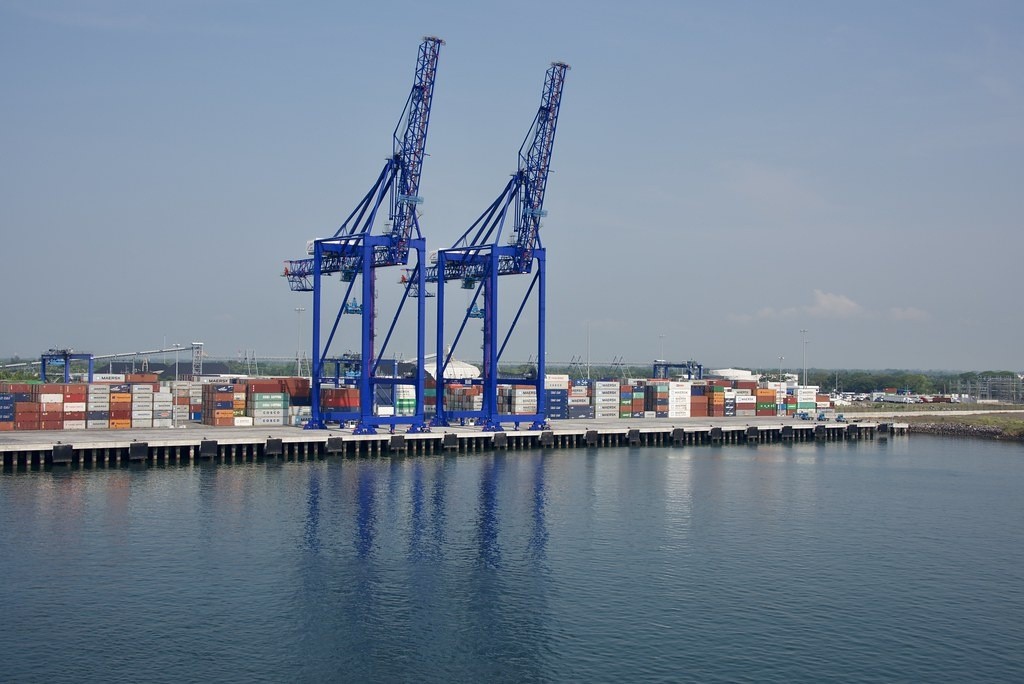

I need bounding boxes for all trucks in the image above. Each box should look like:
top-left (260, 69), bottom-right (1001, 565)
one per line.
top-left (801, 412), bottom-right (814, 420)
top-left (835, 414), bottom-right (846, 422)
top-left (817, 414), bottom-right (829, 421)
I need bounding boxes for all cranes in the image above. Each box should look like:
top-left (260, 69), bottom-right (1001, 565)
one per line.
top-left (280, 36), bottom-right (445, 434)
top-left (397, 62), bottom-right (571, 432)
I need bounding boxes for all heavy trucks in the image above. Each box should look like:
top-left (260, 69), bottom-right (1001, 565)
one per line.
top-left (844, 392), bottom-right (961, 404)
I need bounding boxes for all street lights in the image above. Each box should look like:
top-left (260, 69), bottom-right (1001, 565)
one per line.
top-left (172, 344), bottom-right (180, 428)
top-left (295, 307), bottom-right (305, 356)
top-left (660, 334), bottom-right (666, 379)
top-left (800, 329), bottom-right (808, 389)
top-left (778, 356), bottom-right (785, 415)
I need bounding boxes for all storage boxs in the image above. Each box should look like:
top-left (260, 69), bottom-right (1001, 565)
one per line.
top-left (0, 374), bottom-right (835, 431)
